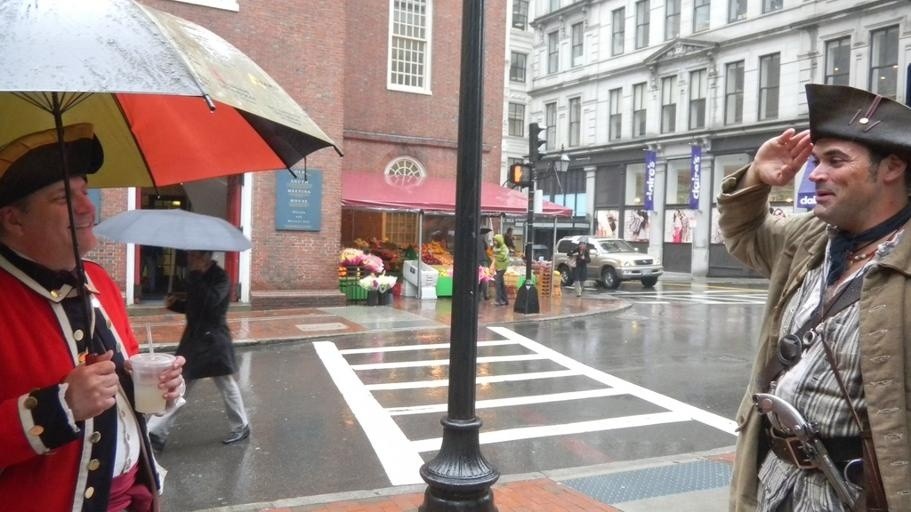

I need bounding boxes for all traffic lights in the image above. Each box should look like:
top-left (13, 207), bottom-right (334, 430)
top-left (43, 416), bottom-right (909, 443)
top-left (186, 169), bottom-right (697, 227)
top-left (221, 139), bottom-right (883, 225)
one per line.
top-left (530, 122), bottom-right (549, 161)
top-left (510, 162), bottom-right (531, 187)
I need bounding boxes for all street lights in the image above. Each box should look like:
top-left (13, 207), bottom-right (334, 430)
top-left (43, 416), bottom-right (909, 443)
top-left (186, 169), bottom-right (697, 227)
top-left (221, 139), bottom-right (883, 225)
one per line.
top-left (547, 143), bottom-right (572, 202)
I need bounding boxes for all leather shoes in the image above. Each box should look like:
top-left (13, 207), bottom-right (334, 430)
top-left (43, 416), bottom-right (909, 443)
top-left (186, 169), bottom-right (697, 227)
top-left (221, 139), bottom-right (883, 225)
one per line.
top-left (223, 425), bottom-right (249, 444)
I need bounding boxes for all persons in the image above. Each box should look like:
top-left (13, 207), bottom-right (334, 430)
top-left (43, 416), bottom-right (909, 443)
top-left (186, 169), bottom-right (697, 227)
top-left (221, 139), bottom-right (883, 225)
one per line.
top-left (147, 249), bottom-right (250, 457)
top-left (714, 82), bottom-right (911, 511)
top-left (567, 243), bottom-right (591, 297)
top-left (479, 225), bottom-right (518, 306)
top-left (0, 123), bottom-right (188, 512)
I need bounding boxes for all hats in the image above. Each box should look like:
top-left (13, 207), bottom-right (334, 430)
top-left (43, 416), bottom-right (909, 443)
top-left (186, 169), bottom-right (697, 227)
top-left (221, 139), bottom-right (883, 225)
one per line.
top-left (804, 82), bottom-right (911, 149)
top-left (0, 122), bottom-right (103, 206)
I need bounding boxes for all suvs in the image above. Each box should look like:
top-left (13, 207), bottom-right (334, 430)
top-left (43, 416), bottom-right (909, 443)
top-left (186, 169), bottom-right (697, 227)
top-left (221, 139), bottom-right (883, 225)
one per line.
top-left (553, 233), bottom-right (665, 291)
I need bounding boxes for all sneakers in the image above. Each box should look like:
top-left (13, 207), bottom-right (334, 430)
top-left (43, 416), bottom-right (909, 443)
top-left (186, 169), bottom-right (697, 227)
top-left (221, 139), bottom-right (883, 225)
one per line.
top-left (149, 431), bottom-right (167, 454)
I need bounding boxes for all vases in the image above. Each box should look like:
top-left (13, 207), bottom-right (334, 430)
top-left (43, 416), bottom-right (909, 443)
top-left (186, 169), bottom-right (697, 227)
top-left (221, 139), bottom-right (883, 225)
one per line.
top-left (367, 289), bottom-right (393, 306)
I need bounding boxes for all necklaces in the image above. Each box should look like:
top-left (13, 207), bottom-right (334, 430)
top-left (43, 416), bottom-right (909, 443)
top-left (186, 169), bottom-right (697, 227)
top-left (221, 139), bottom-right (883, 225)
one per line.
top-left (841, 199), bottom-right (911, 270)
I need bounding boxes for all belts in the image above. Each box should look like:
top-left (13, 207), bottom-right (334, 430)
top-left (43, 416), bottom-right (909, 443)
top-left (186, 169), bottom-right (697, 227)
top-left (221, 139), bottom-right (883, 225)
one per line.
top-left (761, 421), bottom-right (868, 473)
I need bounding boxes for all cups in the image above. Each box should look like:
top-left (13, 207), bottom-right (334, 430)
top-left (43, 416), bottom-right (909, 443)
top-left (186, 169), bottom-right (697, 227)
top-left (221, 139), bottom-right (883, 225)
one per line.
top-left (130, 353), bottom-right (176, 415)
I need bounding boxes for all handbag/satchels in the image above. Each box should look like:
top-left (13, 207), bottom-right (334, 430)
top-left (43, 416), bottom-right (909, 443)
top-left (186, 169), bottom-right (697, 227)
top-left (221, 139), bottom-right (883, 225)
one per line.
top-left (565, 255), bottom-right (577, 268)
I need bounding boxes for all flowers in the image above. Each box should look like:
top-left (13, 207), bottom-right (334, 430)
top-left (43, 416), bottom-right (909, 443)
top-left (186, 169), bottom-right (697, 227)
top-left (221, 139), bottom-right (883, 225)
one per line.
top-left (341, 247), bottom-right (397, 288)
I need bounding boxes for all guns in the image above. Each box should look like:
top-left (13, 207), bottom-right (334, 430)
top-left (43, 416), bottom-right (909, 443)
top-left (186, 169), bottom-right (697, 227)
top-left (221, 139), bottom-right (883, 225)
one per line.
top-left (748, 391), bottom-right (859, 512)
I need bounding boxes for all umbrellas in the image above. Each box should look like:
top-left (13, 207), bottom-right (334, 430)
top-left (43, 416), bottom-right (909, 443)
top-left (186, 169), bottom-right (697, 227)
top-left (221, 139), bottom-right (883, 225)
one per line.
top-left (0, 0), bottom-right (346, 369)
top-left (94, 207), bottom-right (253, 299)
top-left (566, 234), bottom-right (592, 255)
